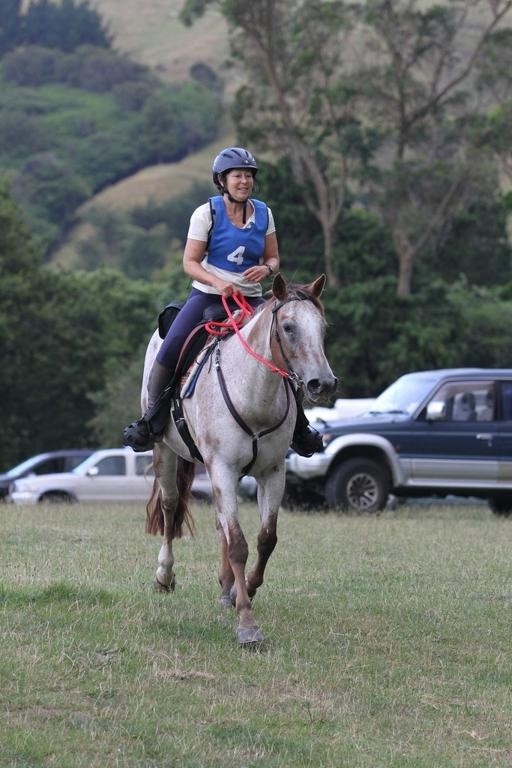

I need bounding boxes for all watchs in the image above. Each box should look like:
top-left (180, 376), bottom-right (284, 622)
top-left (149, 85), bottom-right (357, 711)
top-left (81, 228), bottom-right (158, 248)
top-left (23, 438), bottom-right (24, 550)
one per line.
top-left (263, 263), bottom-right (274, 274)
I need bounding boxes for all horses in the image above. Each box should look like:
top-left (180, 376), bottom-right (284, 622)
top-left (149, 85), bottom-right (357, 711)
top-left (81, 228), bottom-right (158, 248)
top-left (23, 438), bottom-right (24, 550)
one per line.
top-left (142, 273), bottom-right (339, 644)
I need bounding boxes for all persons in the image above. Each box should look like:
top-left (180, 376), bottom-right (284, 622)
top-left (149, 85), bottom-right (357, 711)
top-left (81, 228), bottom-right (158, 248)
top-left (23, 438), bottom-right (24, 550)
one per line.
top-left (124, 148), bottom-right (323, 453)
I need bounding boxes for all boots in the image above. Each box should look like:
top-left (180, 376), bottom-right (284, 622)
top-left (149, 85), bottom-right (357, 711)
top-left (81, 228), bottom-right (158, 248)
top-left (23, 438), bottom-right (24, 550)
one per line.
top-left (292, 425), bottom-right (323, 453)
top-left (124, 361), bottom-right (175, 445)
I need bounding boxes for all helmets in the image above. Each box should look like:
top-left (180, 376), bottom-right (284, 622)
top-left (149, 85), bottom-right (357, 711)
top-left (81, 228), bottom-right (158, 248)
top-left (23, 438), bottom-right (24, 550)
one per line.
top-left (211, 146), bottom-right (259, 175)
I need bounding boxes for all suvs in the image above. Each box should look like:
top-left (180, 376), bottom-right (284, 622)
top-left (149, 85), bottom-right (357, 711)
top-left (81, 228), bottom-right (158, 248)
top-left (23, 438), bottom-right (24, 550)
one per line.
top-left (239, 367), bottom-right (512, 520)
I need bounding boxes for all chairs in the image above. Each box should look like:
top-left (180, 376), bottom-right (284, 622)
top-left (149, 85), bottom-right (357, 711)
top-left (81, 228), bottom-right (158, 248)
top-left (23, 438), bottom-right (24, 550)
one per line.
top-left (454, 386), bottom-right (496, 422)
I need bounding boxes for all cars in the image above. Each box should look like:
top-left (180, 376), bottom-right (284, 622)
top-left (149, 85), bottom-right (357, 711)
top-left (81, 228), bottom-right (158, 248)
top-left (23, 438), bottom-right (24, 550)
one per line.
top-left (0, 448), bottom-right (97, 501)
top-left (7, 444), bottom-right (215, 510)
